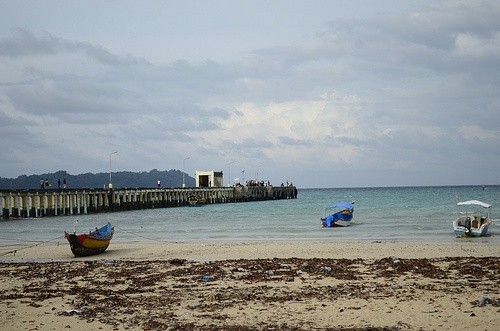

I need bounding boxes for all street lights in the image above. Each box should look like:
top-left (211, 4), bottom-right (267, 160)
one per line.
top-left (182, 158), bottom-right (191, 189)
top-left (109, 151), bottom-right (119, 189)
top-left (228, 161), bottom-right (235, 187)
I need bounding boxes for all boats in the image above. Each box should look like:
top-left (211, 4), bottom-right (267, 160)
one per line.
top-left (64, 221), bottom-right (116, 256)
top-left (318, 203), bottom-right (355, 228)
top-left (452, 199), bottom-right (493, 238)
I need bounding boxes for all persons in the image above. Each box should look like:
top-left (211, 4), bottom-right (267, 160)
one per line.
top-left (246, 179), bottom-right (270, 186)
top-left (57, 178), bottom-right (66, 189)
top-left (40, 178), bottom-right (49, 189)
top-left (157, 179), bottom-right (161, 187)
